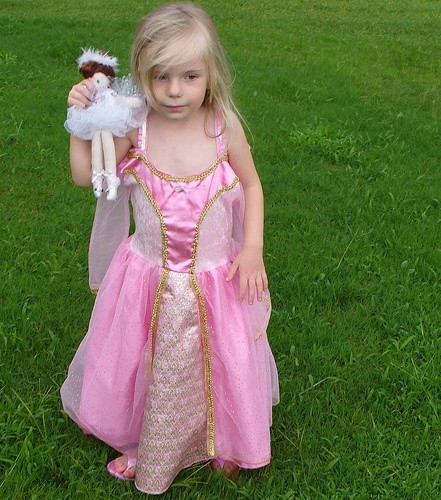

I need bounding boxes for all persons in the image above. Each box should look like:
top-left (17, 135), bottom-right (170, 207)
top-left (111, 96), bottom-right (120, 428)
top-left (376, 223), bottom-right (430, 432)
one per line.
top-left (62, 46), bottom-right (143, 200)
top-left (58, 1), bottom-right (281, 497)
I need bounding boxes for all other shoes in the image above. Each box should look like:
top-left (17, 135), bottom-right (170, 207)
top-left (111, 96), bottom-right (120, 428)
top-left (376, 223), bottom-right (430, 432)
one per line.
top-left (106, 448), bottom-right (138, 481)
top-left (210, 458), bottom-right (240, 479)
